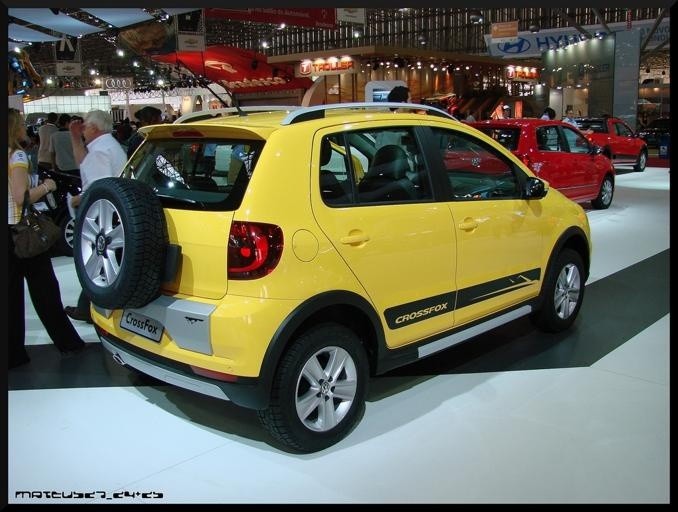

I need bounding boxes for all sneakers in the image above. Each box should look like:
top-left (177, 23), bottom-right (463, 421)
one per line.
top-left (61, 342), bottom-right (101, 359)
top-left (65, 304), bottom-right (92, 324)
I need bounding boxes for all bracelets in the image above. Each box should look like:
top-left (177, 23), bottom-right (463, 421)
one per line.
top-left (42, 183), bottom-right (50, 193)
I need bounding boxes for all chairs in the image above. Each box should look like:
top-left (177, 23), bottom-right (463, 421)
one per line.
top-left (316, 137), bottom-right (420, 201)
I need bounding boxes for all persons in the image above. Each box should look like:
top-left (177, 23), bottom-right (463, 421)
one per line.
top-left (465, 110), bottom-right (478, 122)
top-left (540, 108), bottom-right (556, 120)
top-left (203, 101), bottom-right (218, 177)
top-left (561, 108), bottom-right (594, 152)
top-left (495, 104), bottom-right (513, 139)
top-left (38, 105), bottom-right (163, 175)
top-left (576, 110), bottom-right (581, 119)
top-left (7, 107), bottom-right (105, 370)
top-left (375, 86), bottom-right (413, 152)
top-left (64, 110), bottom-right (128, 324)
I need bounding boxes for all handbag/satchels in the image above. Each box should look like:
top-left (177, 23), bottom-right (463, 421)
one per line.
top-left (11, 211), bottom-right (60, 260)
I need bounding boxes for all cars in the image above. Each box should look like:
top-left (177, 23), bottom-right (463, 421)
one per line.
top-left (442, 119), bottom-right (614, 208)
top-left (37, 169), bottom-right (81, 256)
top-left (634, 115), bottom-right (668, 148)
top-left (71, 102), bottom-right (591, 455)
top-left (574, 116), bottom-right (648, 171)
